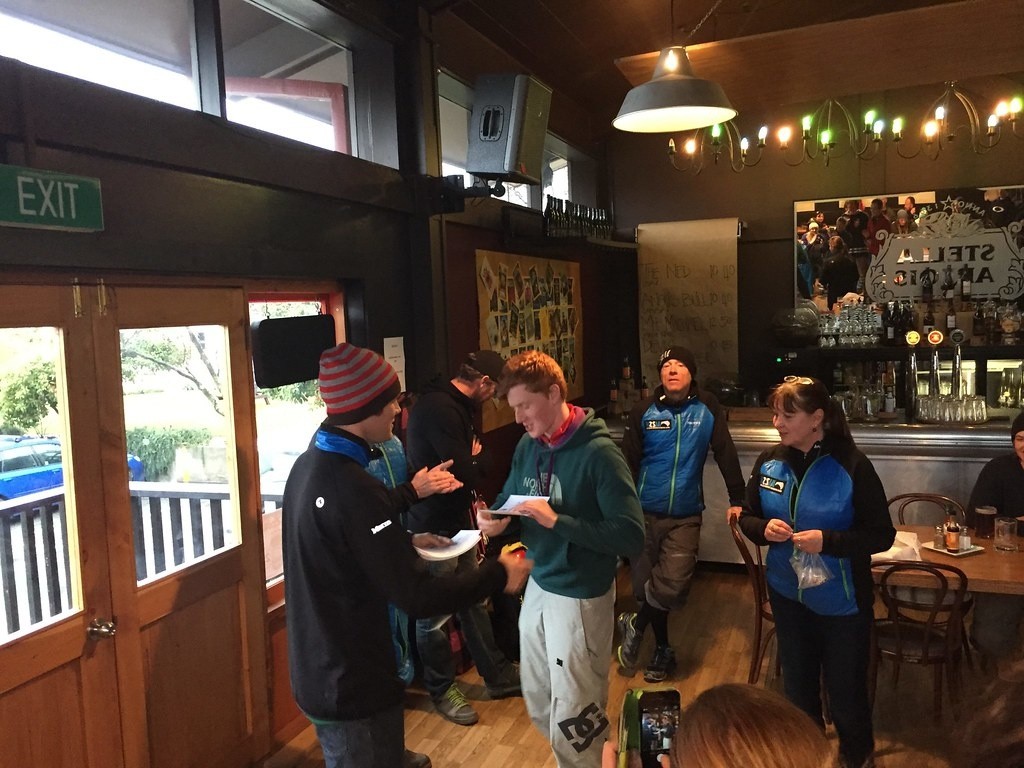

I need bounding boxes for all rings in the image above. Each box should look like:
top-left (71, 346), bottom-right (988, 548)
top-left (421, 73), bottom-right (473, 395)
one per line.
top-left (798, 536), bottom-right (801, 542)
top-left (798, 544), bottom-right (801, 550)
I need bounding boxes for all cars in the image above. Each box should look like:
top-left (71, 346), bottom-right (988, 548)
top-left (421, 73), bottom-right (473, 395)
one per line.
top-left (0, 435), bottom-right (145, 522)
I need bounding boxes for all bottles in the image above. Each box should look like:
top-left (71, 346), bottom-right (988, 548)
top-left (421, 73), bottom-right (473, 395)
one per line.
top-left (622, 357), bottom-right (631, 380)
top-left (642, 376), bottom-right (648, 400)
top-left (921, 263), bottom-right (971, 302)
top-left (934, 501), bottom-right (971, 553)
top-left (544, 194), bottom-right (610, 239)
top-left (829, 378), bottom-right (895, 422)
top-left (610, 379), bottom-right (617, 403)
top-left (973, 295), bottom-right (1023, 346)
top-left (882, 302), bottom-right (956, 346)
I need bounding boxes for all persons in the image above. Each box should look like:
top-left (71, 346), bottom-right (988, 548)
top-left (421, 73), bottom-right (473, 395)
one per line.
top-left (603, 683), bottom-right (832, 768)
top-left (951, 189), bottom-right (1024, 249)
top-left (965, 412), bottom-right (1024, 657)
top-left (797, 196), bottom-right (920, 311)
top-left (738, 375), bottom-right (898, 768)
top-left (365, 431), bottom-right (409, 690)
top-left (404, 350), bottom-right (523, 725)
top-left (476, 349), bottom-right (645, 768)
top-left (616, 347), bottom-right (745, 682)
top-left (282, 341), bottom-right (535, 768)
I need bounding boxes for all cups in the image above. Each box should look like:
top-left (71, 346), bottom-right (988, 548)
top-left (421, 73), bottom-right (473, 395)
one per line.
top-left (975, 506), bottom-right (997, 539)
top-left (818, 305), bottom-right (880, 348)
top-left (916, 393), bottom-right (988, 422)
top-left (993, 517), bottom-right (1018, 554)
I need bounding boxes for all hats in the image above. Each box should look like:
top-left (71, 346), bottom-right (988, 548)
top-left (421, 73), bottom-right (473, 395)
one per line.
top-left (1011, 411), bottom-right (1024, 441)
top-left (467, 350), bottom-right (506, 382)
top-left (657, 346), bottom-right (696, 378)
top-left (319, 343), bottom-right (401, 425)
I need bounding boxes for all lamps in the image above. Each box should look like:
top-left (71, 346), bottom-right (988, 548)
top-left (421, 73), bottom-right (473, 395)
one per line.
top-left (1009, 94), bottom-right (1024, 139)
top-left (778, 98), bottom-right (883, 167)
top-left (892, 79), bottom-right (1007, 161)
top-left (610, 0), bottom-right (739, 133)
top-left (669, 79), bottom-right (769, 176)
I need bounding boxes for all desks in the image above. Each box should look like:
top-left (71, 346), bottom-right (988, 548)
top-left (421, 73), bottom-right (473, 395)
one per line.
top-left (871, 524), bottom-right (1024, 698)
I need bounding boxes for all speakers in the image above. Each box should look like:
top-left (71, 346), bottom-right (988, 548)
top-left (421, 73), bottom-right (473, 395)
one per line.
top-left (250, 314), bottom-right (336, 389)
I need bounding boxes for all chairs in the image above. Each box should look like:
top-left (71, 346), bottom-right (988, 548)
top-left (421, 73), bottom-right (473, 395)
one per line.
top-left (730, 513), bottom-right (833, 726)
top-left (870, 492), bottom-right (975, 723)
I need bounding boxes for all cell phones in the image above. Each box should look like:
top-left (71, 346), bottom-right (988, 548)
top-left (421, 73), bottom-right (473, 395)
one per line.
top-left (620, 687), bottom-right (682, 768)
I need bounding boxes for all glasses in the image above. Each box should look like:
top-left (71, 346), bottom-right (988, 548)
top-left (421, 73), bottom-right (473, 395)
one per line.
top-left (783, 376), bottom-right (814, 386)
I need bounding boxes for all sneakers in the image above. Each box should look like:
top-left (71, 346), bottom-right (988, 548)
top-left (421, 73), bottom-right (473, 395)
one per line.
top-left (645, 646), bottom-right (677, 682)
top-left (487, 664), bottom-right (520, 700)
top-left (617, 612), bottom-right (643, 670)
top-left (435, 682), bottom-right (478, 725)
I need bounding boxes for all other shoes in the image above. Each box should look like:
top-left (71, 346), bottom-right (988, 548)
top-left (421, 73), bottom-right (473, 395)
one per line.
top-left (836, 750), bottom-right (873, 767)
top-left (404, 749), bottom-right (432, 768)
